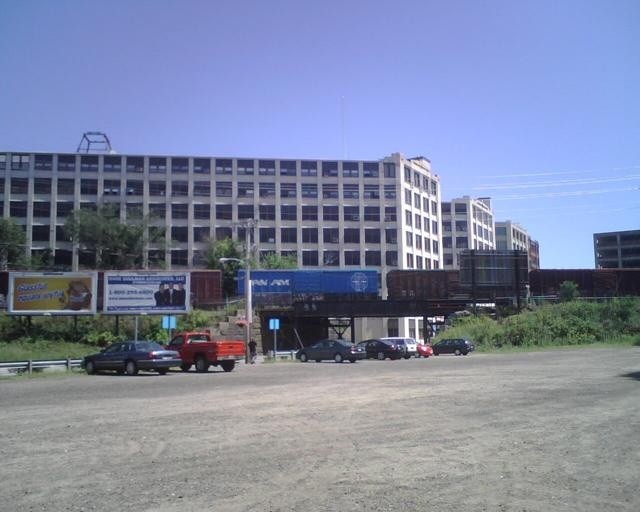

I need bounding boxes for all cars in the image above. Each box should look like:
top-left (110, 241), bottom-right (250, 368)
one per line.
top-left (296, 338), bottom-right (368, 363)
top-left (357, 339), bottom-right (406, 360)
top-left (416, 343), bottom-right (433, 358)
top-left (80, 339), bottom-right (182, 376)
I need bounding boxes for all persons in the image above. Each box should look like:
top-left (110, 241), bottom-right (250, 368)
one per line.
top-left (248, 336), bottom-right (257, 364)
top-left (154, 281), bottom-right (185, 307)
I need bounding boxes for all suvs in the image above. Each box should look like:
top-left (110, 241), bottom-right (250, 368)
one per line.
top-left (430, 337), bottom-right (475, 356)
top-left (381, 337), bottom-right (417, 359)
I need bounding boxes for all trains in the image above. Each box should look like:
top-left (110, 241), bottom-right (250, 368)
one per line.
top-left (191, 266), bottom-right (640, 305)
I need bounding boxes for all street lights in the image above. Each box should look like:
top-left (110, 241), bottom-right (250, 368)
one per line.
top-left (217, 257), bottom-right (251, 364)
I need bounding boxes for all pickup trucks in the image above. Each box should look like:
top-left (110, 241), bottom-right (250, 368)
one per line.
top-left (161, 332), bottom-right (247, 373)
